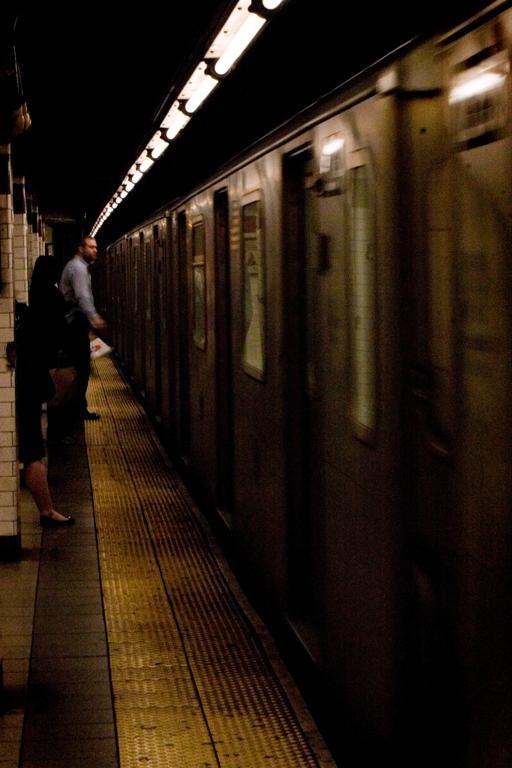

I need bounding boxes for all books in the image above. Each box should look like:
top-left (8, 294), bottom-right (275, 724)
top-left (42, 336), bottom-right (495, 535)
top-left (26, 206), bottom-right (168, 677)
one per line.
top-left (89, 337), bottom-right (111, 361)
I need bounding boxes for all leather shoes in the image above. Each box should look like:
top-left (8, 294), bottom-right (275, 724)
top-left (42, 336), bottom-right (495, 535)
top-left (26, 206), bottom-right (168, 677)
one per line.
top-left (84, 412), bottom-right (101, 420)
top-left (40, 513), bottom-right (75, 527)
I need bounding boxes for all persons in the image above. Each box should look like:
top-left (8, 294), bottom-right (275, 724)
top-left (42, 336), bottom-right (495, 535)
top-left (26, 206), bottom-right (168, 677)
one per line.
top-left (62, 234), bottom-right (102, 420)
top-left (30, 254), bottom-right (96, 446)
top-left (15, 302), bottom-right (77, 527)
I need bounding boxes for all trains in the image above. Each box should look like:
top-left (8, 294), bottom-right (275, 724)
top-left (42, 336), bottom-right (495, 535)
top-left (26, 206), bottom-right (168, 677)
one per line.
top-left (105, 0), bottom-right (512, 768)
